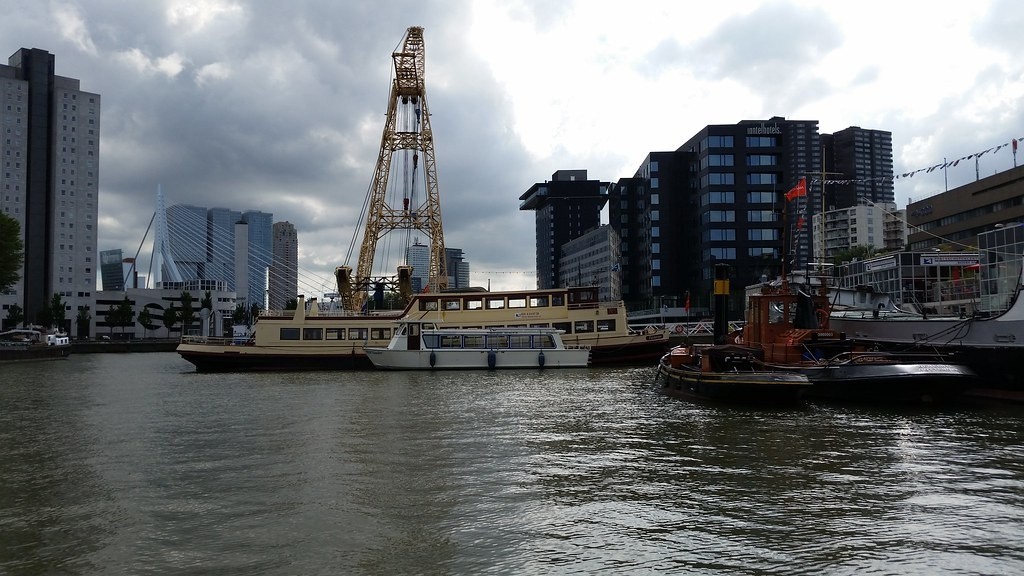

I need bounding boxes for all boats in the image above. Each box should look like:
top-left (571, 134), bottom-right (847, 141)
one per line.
top-left (658, 339), bottom-right (814, 412)
top-left (727, 251), bottom-right (980, 414)
top-left (0, 327), bottom-right (71, 360)
top-left (362, 316), bottom-right (593, 372)
top-left (175, 284), bottom-right (674, 372)
top-left (763, 254), bottom-right (1024, 404)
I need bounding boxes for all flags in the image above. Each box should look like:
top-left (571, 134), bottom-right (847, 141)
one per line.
top-left (685, 293), bottom-right (689, 313)
top-left (787, 177), bottom-right (806, 200)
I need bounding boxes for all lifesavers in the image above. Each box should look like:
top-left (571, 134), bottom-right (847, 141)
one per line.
top-left (816, 309), bottom-right (829, 328)
top-left (675, 325), bottom-right (683, 333)
